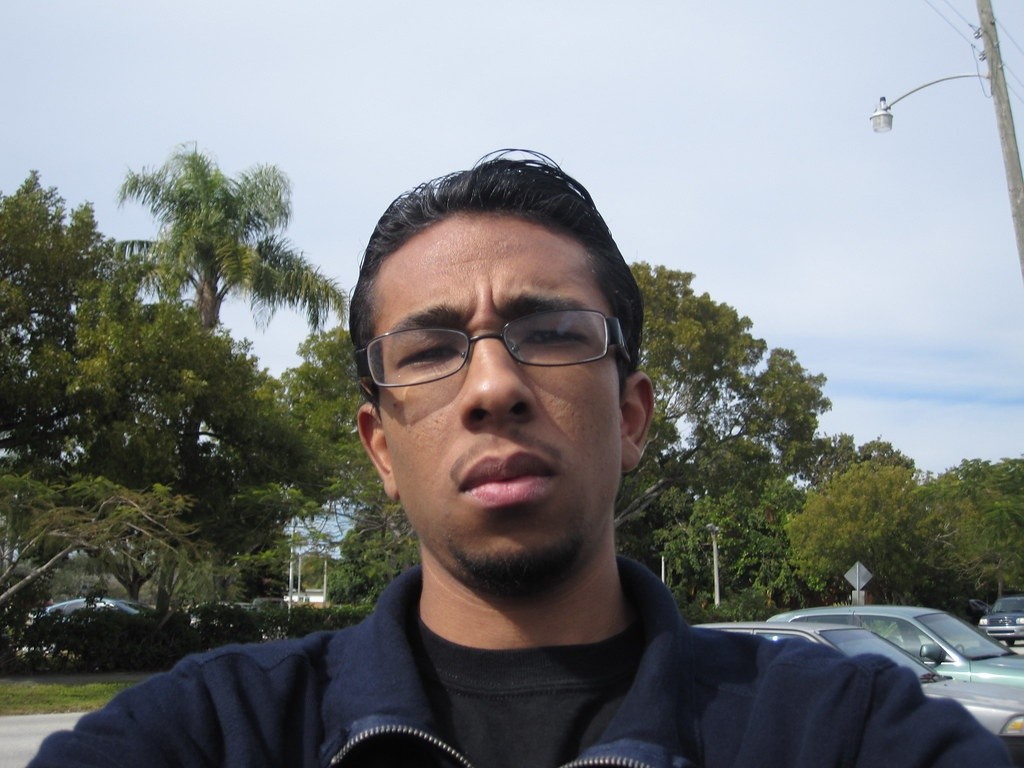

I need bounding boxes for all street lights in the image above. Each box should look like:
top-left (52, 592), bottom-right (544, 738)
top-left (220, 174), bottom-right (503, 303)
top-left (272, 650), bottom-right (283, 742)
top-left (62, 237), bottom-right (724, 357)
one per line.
top-left (704, 522), bottom-right (723, 609)
top-left (867, 72), bottom-right (1024, 281)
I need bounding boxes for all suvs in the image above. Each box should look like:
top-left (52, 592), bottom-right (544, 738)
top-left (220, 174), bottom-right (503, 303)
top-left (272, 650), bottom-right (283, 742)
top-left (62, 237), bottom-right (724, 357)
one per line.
top-left (977, 595), bottom-right (1024, 646)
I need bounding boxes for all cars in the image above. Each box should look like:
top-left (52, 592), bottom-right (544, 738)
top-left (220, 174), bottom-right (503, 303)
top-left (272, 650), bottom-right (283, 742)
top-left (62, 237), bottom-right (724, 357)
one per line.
top-left (690, 620), bottom-right (1024, 768)
top-left (40, 593), bottom-right (288, 630)
top-left (766, 603), bottom-right (1024, 688)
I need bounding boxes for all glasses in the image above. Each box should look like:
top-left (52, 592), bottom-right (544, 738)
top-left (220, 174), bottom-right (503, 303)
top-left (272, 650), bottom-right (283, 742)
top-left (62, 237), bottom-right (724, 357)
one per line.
top-left (354, 308), bottom-right (631, 387)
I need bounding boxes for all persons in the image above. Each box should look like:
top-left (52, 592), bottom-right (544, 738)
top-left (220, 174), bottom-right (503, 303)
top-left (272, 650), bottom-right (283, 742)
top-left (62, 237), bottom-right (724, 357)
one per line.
top-left (22, 148), bottom-right (1016, 768)
